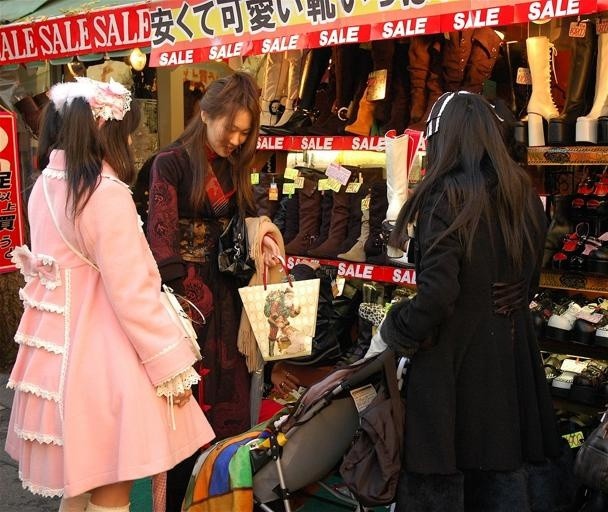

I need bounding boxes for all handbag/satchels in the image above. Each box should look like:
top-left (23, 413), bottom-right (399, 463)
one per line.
top-left (338, 396), bottom-right (405, 508)
top-left (242, 256), bottom-right (321, 361)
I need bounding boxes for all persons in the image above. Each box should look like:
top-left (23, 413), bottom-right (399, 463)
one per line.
top-left (363, 87), bottom-right (572, 509)
top-left (2, 77), bottom-right (217, 510)
top-left (144, 70), bottom-right (284, 512)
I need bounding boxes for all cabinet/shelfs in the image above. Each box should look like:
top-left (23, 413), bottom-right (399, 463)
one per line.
top-left (158, 18), bottom-right (608, 427)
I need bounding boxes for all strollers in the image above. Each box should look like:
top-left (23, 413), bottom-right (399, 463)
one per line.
top-left (200, 346), bottom-right (398, 511)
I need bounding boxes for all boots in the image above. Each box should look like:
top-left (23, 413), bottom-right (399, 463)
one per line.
top-left (258, 10), bottom-right (608, 151)
top-left (281, 264), bottom-right (411, 371)
top-left (254, 130), bottom-right (412, 267)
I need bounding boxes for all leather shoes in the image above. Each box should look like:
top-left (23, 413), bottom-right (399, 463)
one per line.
top-left (549, 166), bottom-right (608, 273)
top-left (527, 293), bottom-right (608, 346)
top-left (539, 349), bottom-right (607, 404)
